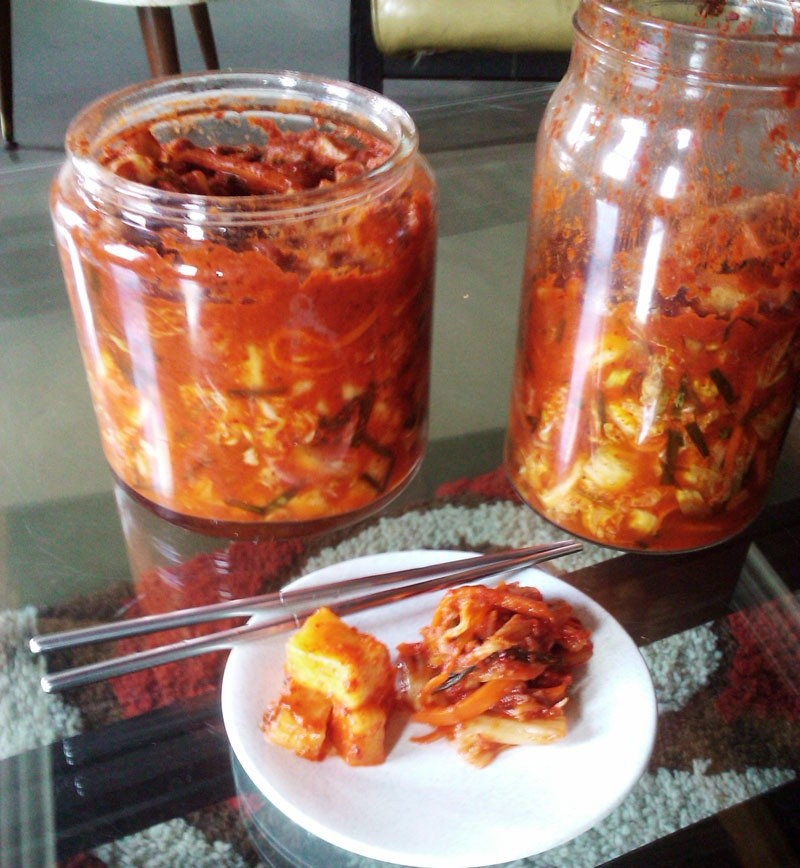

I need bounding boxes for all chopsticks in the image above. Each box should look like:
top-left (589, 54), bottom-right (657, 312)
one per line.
top-left (30, 537), bottom-right (582, 693)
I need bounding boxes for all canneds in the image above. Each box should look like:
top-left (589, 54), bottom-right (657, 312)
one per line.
top-left (46, 72), bottom-right (435, 539)
top-left (501, 1), bottom-right (800, 558)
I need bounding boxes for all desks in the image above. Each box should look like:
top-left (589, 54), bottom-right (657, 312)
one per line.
top-left (0, 104), bottom-right (800, 868)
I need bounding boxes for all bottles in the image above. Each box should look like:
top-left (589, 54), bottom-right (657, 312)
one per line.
top-left (501, 2), bottom-right (800, 560)
top-left (49, 69), bottom-right (435, 540)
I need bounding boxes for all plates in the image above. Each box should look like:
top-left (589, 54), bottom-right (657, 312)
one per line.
top-left (220, 548), bottom-right (657, 868)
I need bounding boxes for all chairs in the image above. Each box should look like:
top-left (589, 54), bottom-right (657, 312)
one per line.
top-left (349, 0), bottom-right (755, 105)
